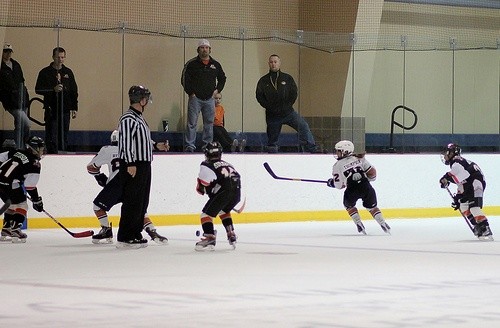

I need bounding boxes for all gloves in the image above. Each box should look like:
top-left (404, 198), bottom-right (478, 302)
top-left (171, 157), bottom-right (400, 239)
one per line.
top-left (31, 196), bottom-right (43, 212)
top-left (327, 179), bottom-right (334, 187)
top-left (440, 172), bottom-right (452, 188)
top-left (451, 194), bottom-right (462, 209)
top-left (94, 173), bottom-right (108, 187)
top-left (196, 183), bottom-right (204, 195)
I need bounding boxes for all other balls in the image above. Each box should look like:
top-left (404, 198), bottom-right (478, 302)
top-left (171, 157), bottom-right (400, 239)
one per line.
top-left (196, 231), bottom-right (200, 236)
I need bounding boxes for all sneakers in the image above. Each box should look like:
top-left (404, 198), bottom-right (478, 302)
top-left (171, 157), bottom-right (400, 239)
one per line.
top-left (146, 226), bottom-right (168, 244)
top-left (195, 230), bottom-right (217, 250)
top-left (356, 218), bottom-right (366, 234)
top-left (116, 238), bottom-right (147, 248)
top-left (92, 222), bottom-right (113, 244)
top-left (381, 221), bottom-right (392, 235)
top-left (477, 224), bottom-right (493, 240)
top-left (0, 221), bottom-right (12, 240)
top-left (227, 225), bottom-right (237, 248)
top-left (10, 222), bottom-right (27, 243)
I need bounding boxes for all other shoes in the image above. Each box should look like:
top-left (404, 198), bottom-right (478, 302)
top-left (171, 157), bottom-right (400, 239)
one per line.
top-left (240, 139), bottom-right (246, 152)
top-left (231, 139), bottom-right (238, 152)
top-left (187, 147), bottom-right (193, 152)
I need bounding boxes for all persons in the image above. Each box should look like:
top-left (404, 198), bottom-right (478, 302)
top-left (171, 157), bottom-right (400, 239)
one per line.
top-left (180, 38), bottom-right (226, 152)
top-left (0, 136), bottom-right (47, 243)
top-left (439, 143), bottom-right (494, 242)
top-left (255, 54), bottom-right (321, 154)
top-left (34, 47), bottom-right (79, 155)
top-left (87, 129), bottom-right (169, 245)
top-left (0, 44), bottom-right (33, 153)
top-left (326, 140), bottom-right (391, 235)
top-left (195, 141), bottom-right (241, 251)
top-left (212, 93), bottom-right (247, 152)
top-left (117, 84), bottom-right (154, 249)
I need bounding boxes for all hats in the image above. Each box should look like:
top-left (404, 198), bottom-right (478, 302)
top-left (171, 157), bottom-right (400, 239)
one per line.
top-left (199, 39), bottom-right (211, 47)
top-left (3, 44), bottom-right (13, 52)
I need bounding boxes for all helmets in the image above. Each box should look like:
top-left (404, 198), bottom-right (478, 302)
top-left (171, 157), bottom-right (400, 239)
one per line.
top-left (27, 136), bottom-right (46, 159)
top-left (441, 143), bottom-right (460, 164)
top-left (129, 85), bottom-right (151, 102)
top-left (111, 130), bottom-right (119, 142)
top-left (333, 140), bottom-right (354, 159)
top-left (204, 142), bottom-right (222, 158)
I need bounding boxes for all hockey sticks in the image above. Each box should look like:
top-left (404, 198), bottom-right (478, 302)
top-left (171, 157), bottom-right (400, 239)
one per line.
top-left (263, 162), bottom-right (328, 183)
top-left (445, 187), bottom-right (474, 234)
top-left (25, 195), bottom-right (94, 238)
top-left (232, 196), bottom-right (246, 214)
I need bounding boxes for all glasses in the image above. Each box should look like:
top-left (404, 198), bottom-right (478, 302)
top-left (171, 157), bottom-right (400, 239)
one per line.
top-left (4, 49), bottom-right (12, 53)
